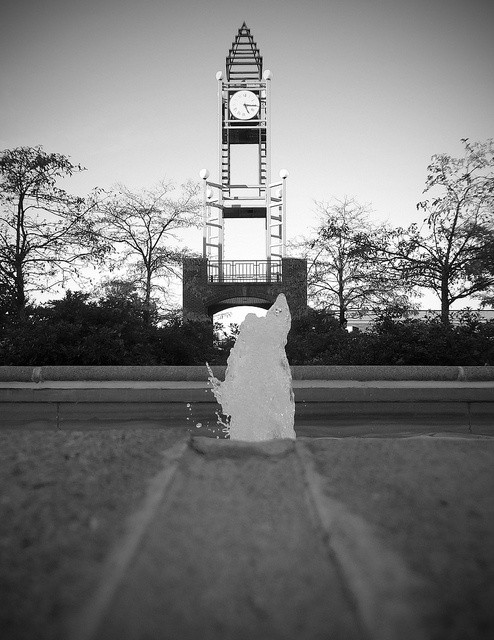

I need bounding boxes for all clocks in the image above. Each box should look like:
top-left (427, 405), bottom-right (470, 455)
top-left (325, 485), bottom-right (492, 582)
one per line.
top-left (227, 88), bottom-right (260, 120)
